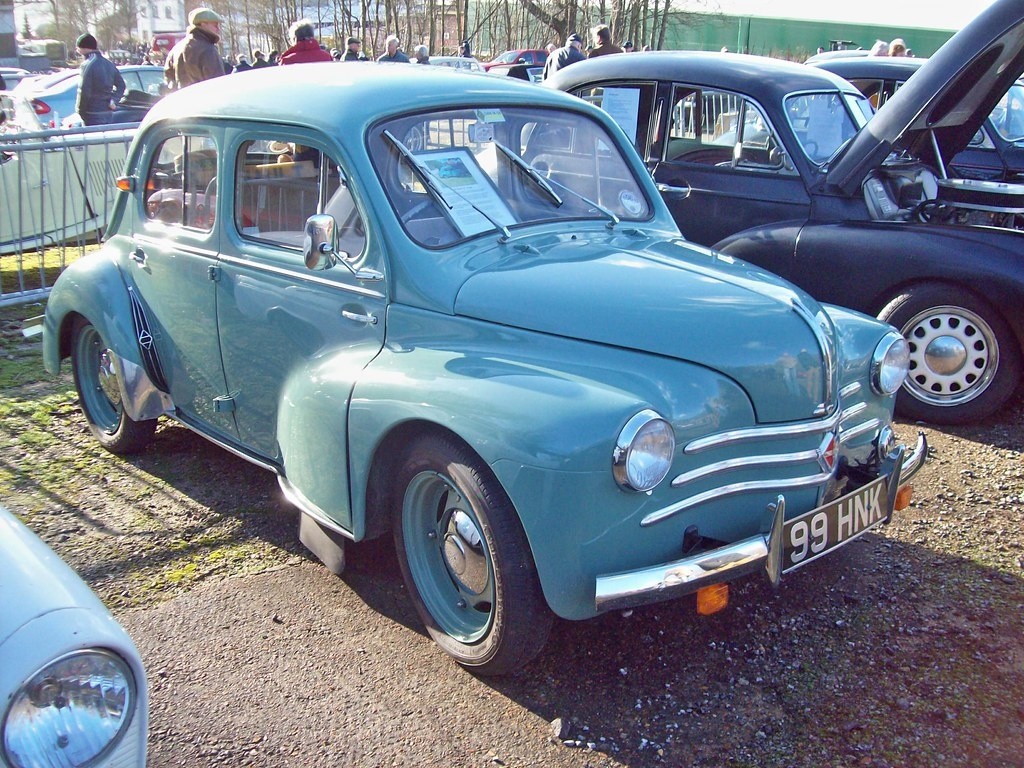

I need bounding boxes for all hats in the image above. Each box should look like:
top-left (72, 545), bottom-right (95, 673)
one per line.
top-left (623, 41), bottom-right (632, 48)
top-left (332, 50), bottom-right (340, 58)
top-left (584, 45), bottom-right (592, 51)
top-left (236, 53), bottom-right (248, 61)
top-left (76, 33), bottom-right (97, 50)
top-left (348, 38), bottom-right (362, 44)
top-left (188, 7), bottom-right (226, 24)
top-left (567, 34), bottom-right (582, 49)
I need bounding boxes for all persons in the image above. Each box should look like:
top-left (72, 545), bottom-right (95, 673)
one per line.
top-left (721, 47), bottom-right (729, 53)
top-left (107, 24), bottom-right (651, 80)
top-left (817, 47), bottom-right (825, 54)
top-left (76, 32), bottom-right (126, 126)
top-left (164, 8), bottom-right (226, 91)
top-left (279, 19), bottom-right (333, 65)
top-left (868, 38), bottom-right (916, 57)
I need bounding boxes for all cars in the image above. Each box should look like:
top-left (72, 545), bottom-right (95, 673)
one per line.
top-left (403, 1), bottom-right (1023, 426)
top-left (0, 49), bottom-right (549, 156)
top-left (37, 61), bottom-right (929, 678)
top-left (714, 50), bottom-right (1024, 176)
